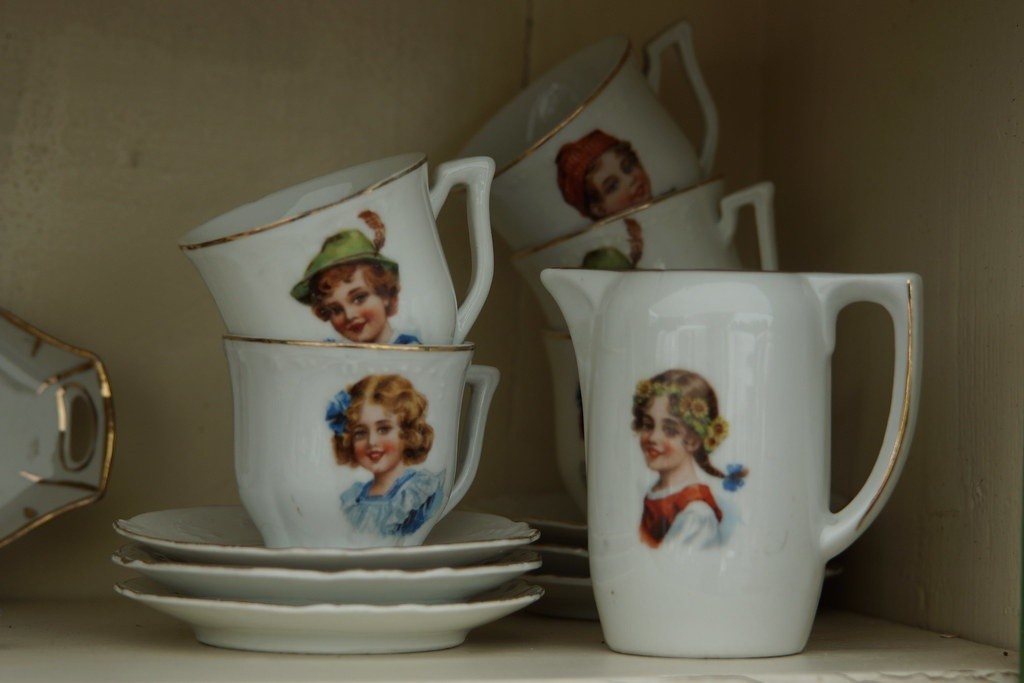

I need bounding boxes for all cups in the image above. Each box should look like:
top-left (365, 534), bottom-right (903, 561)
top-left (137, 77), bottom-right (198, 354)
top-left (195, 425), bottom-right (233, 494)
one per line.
top-left (177, 150), bottom-right (495, 345)
top-left (458, 21), bottom-right (778, 520)
top-left (223, 335), bottom-right (502, 550)
top-left (539, 266), bottom-right (924, 660)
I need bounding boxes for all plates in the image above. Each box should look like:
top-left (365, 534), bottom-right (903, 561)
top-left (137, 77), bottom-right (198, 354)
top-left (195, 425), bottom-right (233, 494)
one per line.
top-left (114, 576), bottom-right (543, 655)
top-left (0, 308), bottom-right (115, 552)
top-left (111, 543), bottom-right (544, 604)
top-left (112, 506), bottom-right (538, 568)
top-left (527, 512), bottom-right (842, 618)
